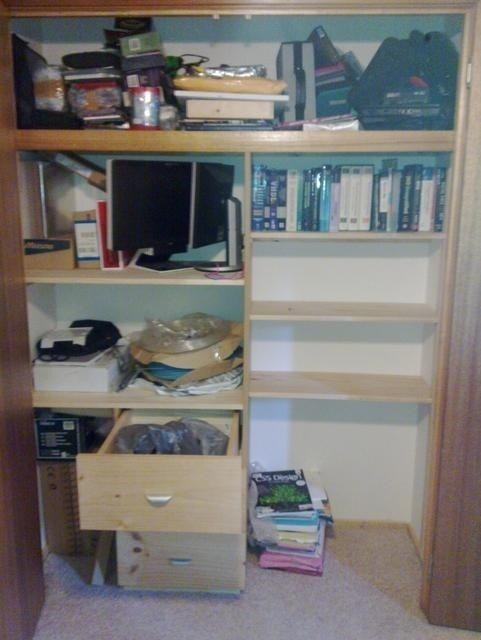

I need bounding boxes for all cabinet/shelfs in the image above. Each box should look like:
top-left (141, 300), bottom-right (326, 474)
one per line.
top-left (10, 14), bottom-right (475, 594)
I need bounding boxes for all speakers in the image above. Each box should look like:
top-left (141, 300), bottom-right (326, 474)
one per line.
top-left (197, 196), bottom-right (244, 271)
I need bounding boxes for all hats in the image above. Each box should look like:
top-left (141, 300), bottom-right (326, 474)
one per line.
top-left (127, 314), bottom-right (245, 369)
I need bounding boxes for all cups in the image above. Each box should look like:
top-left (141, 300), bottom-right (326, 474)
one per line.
top-left (129, 87), bottom-right (162, 130)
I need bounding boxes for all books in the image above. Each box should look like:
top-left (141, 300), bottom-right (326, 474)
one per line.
top-left (250, 466), bottom-right (332, 578)
top-left (173, 89), bottom-right (290, 132)
top-left (32, 334), bottom-right (133, 394)
top-left (254, 157), bottom-right (448, 231)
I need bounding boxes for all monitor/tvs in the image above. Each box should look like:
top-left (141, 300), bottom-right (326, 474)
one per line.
top-left (104, 158), bottom-right (235, 272)
